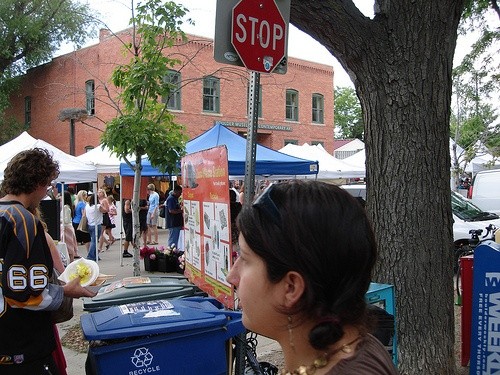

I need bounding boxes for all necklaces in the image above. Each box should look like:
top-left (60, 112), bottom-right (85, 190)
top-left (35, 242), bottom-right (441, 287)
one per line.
top-left (282, 334), bottom-right (362, 375)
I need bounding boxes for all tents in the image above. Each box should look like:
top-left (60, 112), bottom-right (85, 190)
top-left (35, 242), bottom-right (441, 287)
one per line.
top-left (0, 131), bottom-right (38, 184)
top-left (119, 123), bottom-right (320, 267)
top-left (0, 138), bottom-right (97, 243)
top-left (276, 136), bottom-right (500, 186)
top-left (74, 141), bottom-right (180, 174)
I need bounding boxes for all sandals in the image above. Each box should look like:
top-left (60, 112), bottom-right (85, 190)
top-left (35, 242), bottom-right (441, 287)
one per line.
top-left (143, 241), bottom-right (152, 244)
top-left (149, 241), bottom-right (158, 245)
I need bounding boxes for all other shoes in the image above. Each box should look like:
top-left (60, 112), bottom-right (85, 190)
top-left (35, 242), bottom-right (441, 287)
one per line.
top-left (95, 256), bottom-right (102, 261)
top-left (123, 253), bottom-right (133, 257)
top-left (108, 239), bottom-right (116, 245)
top-left (105, 243), bottom-right (110, 251)
top-left (74, 256), bottom-right (83, 259)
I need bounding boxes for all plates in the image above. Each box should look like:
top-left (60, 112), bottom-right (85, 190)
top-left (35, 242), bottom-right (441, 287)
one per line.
top-left (57, 258), bottom-right (99, 288)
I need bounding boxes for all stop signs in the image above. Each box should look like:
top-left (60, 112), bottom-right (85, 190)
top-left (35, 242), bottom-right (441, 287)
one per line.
top-left (231, 0), bottom-right (288, 74)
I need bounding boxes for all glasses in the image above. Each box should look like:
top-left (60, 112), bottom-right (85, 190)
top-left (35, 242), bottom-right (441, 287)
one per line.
top-left (45, 184), bottom-right (54, 192)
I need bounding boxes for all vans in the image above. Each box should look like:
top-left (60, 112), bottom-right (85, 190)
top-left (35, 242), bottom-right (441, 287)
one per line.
top-left (467, 169), bottom-right (500, 212)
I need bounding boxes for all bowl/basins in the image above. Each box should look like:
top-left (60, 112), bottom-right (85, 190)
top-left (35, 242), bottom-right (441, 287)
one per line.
top-left (85, 281), bottom-right (103, 293)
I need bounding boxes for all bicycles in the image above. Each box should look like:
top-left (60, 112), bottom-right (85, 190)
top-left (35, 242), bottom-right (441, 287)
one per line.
top-left (457, 224), bottom-right (497, 296)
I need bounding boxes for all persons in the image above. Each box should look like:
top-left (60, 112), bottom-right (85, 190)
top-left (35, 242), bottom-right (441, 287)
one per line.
top-left (105, 195), bottom-right (116, 245)
top-left (112, 184), bottom-right (120, 201)
top-left (66, 187), bottom-right (76, 206)
top-left (165, 185), bottom-right (183, 249)
top-left (233, 181), bottom-right (240, 189)
top-left (0, 182), bottom-right (68, 375)
top-left (0, 147), bottom-right (97, 375)
top-left (146, 183), bottom-right (160, 245)
top-left (139, 199), bottom-right (149, 247)
top-left (82, 194), bottom-right (107, 262)
top-left (226, 179), bottom-right (399, 375)
top-left (97, 189), bottom-right (110, 254)
top-left (229, 190), bottom-right (242, 224)
top-left (72, 190), bottom-right (92, 254)
top-left (121, 199), bottom-right (133, 257)
top-left (58, 191), bottom-right (82, 265)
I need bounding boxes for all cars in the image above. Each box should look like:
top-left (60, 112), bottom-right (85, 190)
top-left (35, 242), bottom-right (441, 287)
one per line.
top-left (340, 184), bottom-right (499, 273)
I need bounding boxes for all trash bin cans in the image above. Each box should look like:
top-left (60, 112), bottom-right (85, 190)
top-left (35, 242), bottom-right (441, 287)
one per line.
top-left (84, 273), bottom-right (205, 309)
top-left (80, 292), bottom-right (245, 375)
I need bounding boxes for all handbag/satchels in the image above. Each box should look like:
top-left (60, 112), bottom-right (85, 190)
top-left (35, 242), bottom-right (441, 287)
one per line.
top-left (77, 216), bottom-right (89, 233)
